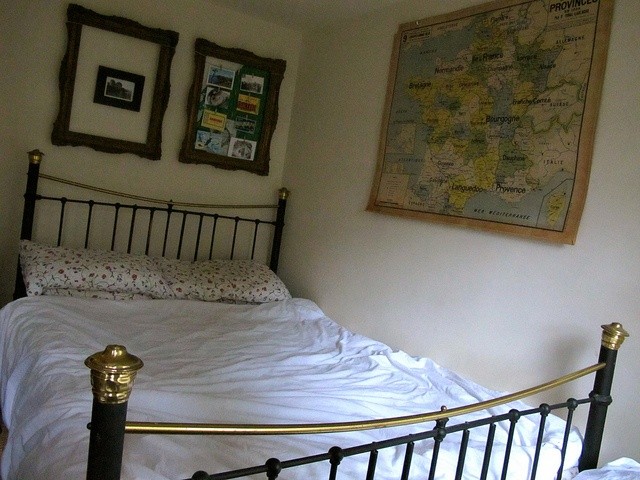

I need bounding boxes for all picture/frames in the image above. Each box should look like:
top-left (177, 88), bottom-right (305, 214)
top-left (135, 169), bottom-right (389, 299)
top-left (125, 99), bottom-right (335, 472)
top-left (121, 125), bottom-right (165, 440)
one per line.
top-left (180, 37), bottom-right (288, 176)
top-left (52, 3), bottom-right (179, 162)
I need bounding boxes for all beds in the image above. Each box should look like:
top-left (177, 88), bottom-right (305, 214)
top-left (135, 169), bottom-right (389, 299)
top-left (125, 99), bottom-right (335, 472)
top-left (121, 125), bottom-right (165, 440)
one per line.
top-left (1, 149), bottom-right (630, 480)
